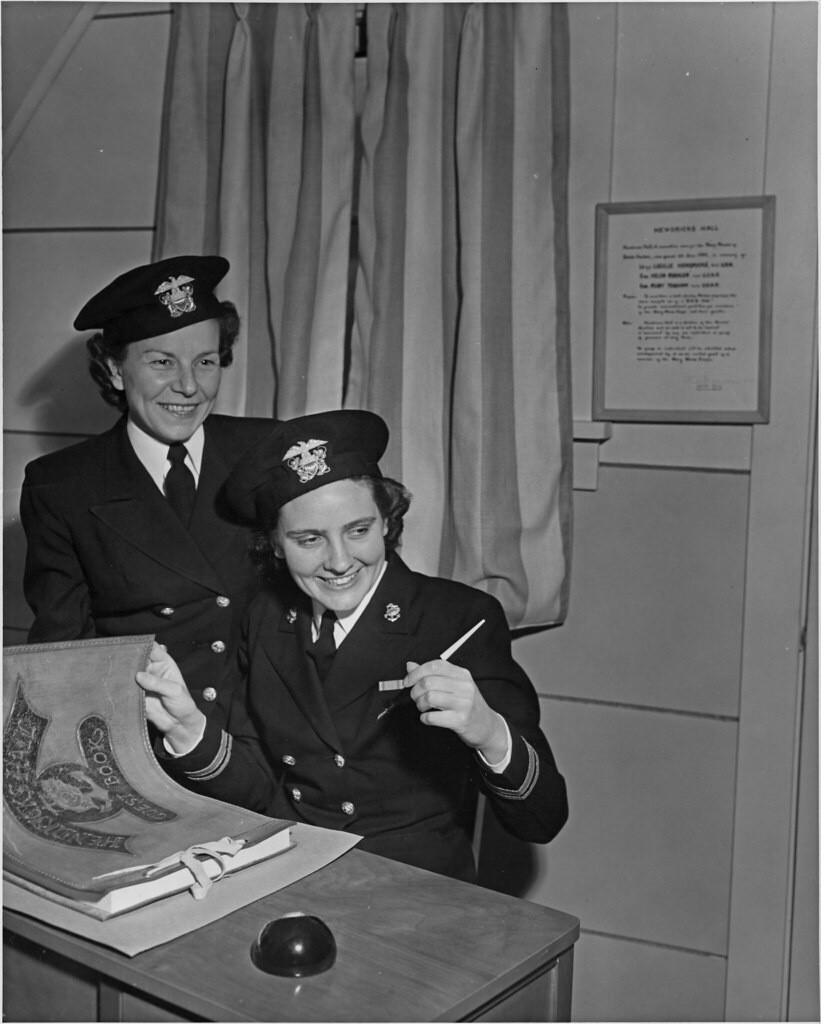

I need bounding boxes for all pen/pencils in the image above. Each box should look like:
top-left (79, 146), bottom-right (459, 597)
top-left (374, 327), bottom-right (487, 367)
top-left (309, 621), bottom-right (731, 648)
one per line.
top-left (374, 617), bottom-right (487, 722)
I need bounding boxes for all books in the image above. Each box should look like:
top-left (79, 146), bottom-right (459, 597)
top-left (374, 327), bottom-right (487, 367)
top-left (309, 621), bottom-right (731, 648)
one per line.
top-left (3, 633), bottom-right (300, 922)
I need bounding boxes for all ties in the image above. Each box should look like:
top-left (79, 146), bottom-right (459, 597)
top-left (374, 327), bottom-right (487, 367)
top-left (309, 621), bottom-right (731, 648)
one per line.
top-left (313, 607), bottom-right (338, 674)
top-left (163, 445), bottom-right (196, 531)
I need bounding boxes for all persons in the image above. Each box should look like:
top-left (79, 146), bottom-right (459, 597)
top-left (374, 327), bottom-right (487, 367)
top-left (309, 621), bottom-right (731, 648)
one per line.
top-left (18, 254), bottom-right (289, 794)
top-left (135, 408), bottom-right (570, 887)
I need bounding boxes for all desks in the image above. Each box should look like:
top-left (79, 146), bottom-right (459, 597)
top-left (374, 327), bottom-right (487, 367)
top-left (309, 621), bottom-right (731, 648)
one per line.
top-left (3, 846), bottom-right (579, 1022)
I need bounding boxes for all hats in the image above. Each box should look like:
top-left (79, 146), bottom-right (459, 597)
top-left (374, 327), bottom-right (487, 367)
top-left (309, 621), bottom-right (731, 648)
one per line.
top-left (72, 254), bottom-right (232, 345)
top-left (223, 409), bottom-right (389, 526)
